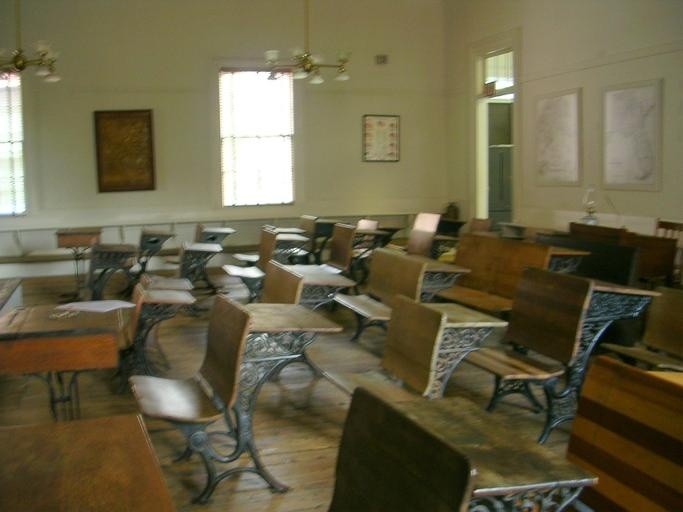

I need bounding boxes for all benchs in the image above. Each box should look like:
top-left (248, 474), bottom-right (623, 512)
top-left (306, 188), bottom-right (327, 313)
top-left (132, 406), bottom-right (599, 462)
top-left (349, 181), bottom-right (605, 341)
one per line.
top-left (200, 225), bottom-right (236, 245)
top-left (435, 236), bottom-right (553, 317)
top-left (568, 346), bottom-right (682, 509)
top-left (602, 285), bottom-right (683, 371)
top-left (179, 241), bottom-right (222, 291)
top-left (298, 213), bottom-right (318, 258)
top-left (233, 223), bottom-right (276, 268)
top-left (223, 227), bottom-right (275, 304)
top-left (328, 250), bottom-right (428, 342)
top-left (130, 292), bottom-right (318, 505)
top-left (300, 294), bottom-right (493, 406)
top-left (89, 231), bottom-right (174, 303)
top-left (655, 217), bottom-right (683, 283)
top-left (462, 267), bottom-right (655, 446)
top-left (404, 228), bottom-right (434, 257)
top-left (260, 259), bottom-right (342, 313)
top-left (315, 223), bottom-right (361, 314)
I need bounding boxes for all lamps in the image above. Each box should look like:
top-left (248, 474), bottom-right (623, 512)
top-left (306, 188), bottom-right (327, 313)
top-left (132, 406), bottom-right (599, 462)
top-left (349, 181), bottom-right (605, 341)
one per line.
top-left (263, 0), bottom-right (350, 85)
top-left (0, 0), bottom-right (66, 84)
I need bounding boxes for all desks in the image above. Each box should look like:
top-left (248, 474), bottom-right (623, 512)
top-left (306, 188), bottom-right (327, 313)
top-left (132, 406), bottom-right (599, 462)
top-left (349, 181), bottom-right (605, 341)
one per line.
top-left (423, 301), bottom-right (509, 331)
top-left (354, 227), bottom-right (387, 253)
top-left (434, 233), bottom-right (465, 260)
top-left (275, 231), bottom-right (309, 263)
top-left (275, 225), bottom-right (308, 235)
top-left (1, 412), bottom-right (177, 512)
top-left (519, 238), bottom-right (591, 275)
top-left (570, 224), bottom-right (678, 288)
top-left (400, 249), bottom-right (470, 302)
top-left (283, 264), bottom-right (357, 287)
top-left (585, 272), bottom-right (665, 299)
top-left (387, 393), bottom-right (600, 512)
top-left (382, 293), bottom-right (510, 400)
top-left (0, 305), bottom-right (139, 421)
top-left (241, 303), bottom-right (343, 334)
top-left (54, 225), bottom-right (104, 304)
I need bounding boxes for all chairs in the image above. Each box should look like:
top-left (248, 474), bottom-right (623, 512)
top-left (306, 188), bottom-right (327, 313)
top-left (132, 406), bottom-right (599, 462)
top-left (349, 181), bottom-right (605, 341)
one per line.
top-left (356, 218), bottom-right (378, 240)
top-left (329, 385), bottom-right (477, 512)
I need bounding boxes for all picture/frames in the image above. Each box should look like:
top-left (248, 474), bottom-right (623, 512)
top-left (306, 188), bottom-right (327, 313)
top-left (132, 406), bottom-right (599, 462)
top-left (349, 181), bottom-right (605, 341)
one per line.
top-left (600, 78), bottom-right (665, 192)
top-left (92, 109), bottom-right (157, 194)
top-left (533, 86), bottom-right (583, 187)
top-left (363, 114), bottom-right (402, 164)
